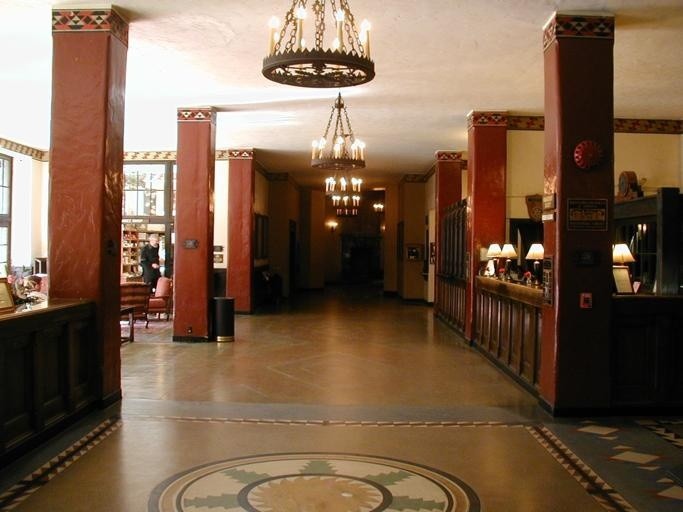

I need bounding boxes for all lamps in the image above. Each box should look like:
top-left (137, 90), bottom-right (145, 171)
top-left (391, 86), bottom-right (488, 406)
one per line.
top-left (485, 243), bottom-right (501, 277)
top-left (310, 91), bottom-right (365, 171)
top-left (261, 0), bottom-right (375, 88)
top-left (324, 169), bottom-right (363, 217)
top-left (612, 243), bottom-right (636, 266)
top-left (499, 244), bottom-right (517, 279)
top-left (525, 244), bottom-right (544, 288)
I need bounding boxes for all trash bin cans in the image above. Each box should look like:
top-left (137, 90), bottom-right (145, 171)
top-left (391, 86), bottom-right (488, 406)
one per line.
top-left (211, 298), bottom-right (234, 342)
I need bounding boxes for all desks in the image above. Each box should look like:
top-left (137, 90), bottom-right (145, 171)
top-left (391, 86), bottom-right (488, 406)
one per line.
top-left (120, 304), bottom-right (137, 345)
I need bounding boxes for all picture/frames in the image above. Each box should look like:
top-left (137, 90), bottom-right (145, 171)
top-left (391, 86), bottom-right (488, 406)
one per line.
top-left (613, 267), bottom-right (635, 295)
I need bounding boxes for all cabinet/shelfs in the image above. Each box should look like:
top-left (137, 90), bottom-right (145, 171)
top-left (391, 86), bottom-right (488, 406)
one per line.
top-left (122, 229), bottom-right (160, 275)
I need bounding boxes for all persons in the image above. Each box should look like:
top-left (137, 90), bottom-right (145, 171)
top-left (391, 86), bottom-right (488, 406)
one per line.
top-left (139, 233), bottom-right (161, 293)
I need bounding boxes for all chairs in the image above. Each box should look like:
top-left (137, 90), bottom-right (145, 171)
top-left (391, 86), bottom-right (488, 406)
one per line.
top-left (119, 274), bottom-right (173, 330)
